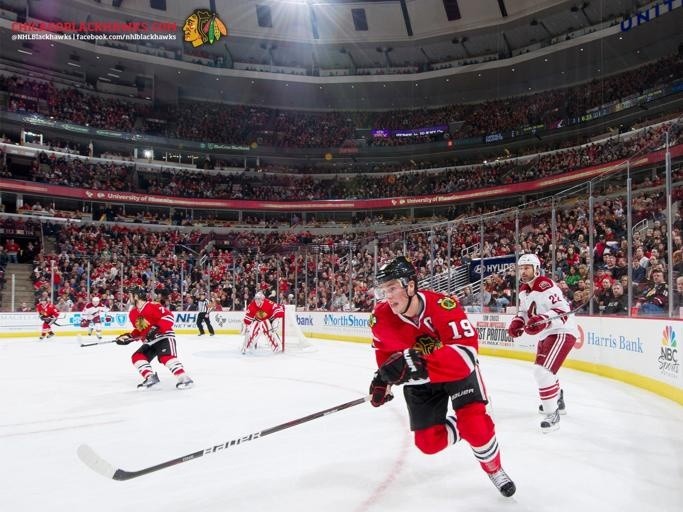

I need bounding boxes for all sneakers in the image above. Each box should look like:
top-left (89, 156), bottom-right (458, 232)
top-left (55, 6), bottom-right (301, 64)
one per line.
top-left (138, 375), bottom-right (159, 386)
top-left (539, 400), bottom-right (565, 427)
top-left (176, 380), bottom-right (193, 386)
top-left (488, 467), bottom-right (515, 497)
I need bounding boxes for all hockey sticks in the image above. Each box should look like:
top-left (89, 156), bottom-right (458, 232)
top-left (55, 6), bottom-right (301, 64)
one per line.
top-left (42, 313), bottom-right (67, 319)
top-left (81, 337), bottom-right (151, 348)
top-left (77, 388), bottom-right (394, 480)
top-left (517, 287), bottom-right (595, 330)
top-left (53, 321), bottom-right (93, 326)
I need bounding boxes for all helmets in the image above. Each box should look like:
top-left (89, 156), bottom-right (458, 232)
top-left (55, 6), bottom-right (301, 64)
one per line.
top-left (517, 254), bottom-right (540, 277)
top-left (376, 256), bottom-right (418, 287)
top-left (254, 292), bottom-right (265, 300)
top-left (123, 286), bottom-right (147, 301)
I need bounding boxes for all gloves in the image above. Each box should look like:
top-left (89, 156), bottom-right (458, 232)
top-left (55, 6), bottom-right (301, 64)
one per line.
top-left (509, 315), bottom-right (550, 337)
top-left (147, 325), bottom-right (159, 339)
top-left (116, 333), bottom-right (133, 344)
top-left (370, 348), bottom-right (429, 407)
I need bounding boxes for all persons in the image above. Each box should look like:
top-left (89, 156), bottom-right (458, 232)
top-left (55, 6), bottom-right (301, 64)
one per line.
top-left (35, 296), bottom-right (61, 339)
top-left (507, 253), bottom-right (580, 430)
top-left (0, 1), bottom-right (682, 317)
top-left (196, 291), bottom-right (215, 337)
top-left (368, 255), bottom-right (517, 498)
top-left (80, 296), bottom-right (112, 339)
top-left (238, 292), bottom-right (284, 353)
top-left (115, 283), bottom-right (194, 388)
